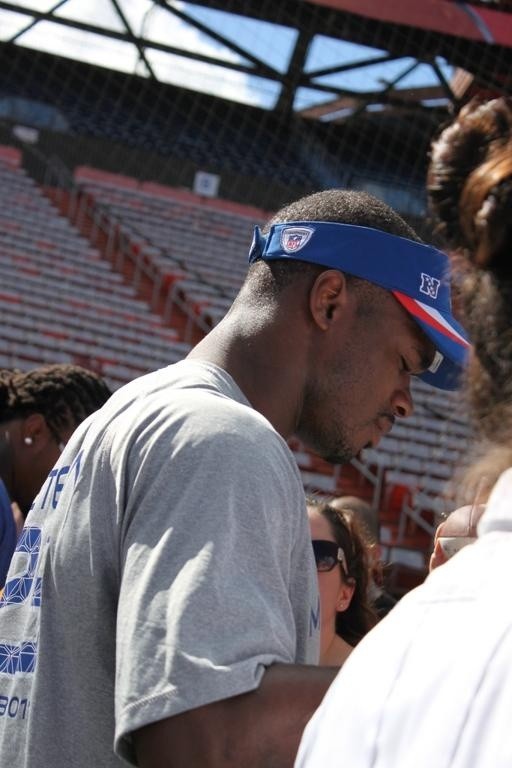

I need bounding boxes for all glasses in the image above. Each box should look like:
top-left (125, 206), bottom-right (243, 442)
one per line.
top-left (311, 540), bottom-right (350, 575)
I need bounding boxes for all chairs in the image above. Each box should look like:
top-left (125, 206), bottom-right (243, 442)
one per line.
top-left (0, 169), bottom-right (479, 589)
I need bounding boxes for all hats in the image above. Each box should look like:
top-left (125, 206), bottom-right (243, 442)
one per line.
top-left (247, 222), bottom-right (475, 392)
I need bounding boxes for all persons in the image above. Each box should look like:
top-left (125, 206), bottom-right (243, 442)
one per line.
top-left (329, 496), bottom-right (397, 620)
top-left (294, 495), bottom-right (380, 667)
top-left (294, 93), bottom-right (512, 767)
top-left (0, 362), bottom-right (113, 591)
top-left (0, 187), bottom-right (472, 768)
